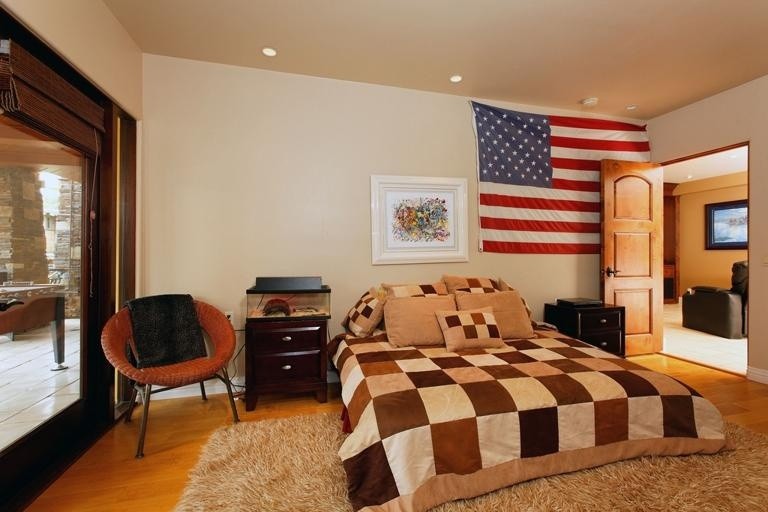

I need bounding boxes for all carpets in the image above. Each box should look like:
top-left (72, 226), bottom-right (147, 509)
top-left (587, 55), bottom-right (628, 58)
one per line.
top-left (168, 411), bottom-right (767, 510)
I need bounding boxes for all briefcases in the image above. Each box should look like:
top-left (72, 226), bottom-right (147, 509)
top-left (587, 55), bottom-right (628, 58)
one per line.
top-left (682, 286), bottom-right (742, 339)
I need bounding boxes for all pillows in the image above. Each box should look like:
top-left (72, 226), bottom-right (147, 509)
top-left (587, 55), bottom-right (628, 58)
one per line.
top-left (339, 273), bottom-right (535, 351)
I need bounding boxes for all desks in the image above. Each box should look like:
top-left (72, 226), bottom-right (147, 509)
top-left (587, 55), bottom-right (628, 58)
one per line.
top-left (0, 282), bottom-right (70, 371)
top-left (663, 264), bottom-right (675, 303)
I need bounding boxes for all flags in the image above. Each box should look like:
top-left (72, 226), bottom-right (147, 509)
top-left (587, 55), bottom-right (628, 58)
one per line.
top-left (469, 98), bottom-right (653, 254)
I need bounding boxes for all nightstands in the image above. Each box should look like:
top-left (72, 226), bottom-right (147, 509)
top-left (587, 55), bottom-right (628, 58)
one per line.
top-left (543, 299), bottom-right (627, 359)
top-left (242, 313), bottom-right (332, 413)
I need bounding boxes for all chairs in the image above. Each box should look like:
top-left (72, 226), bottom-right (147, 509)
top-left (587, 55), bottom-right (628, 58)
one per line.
top-left (98, 292), bottom-right (240, 460)
top-left (681, 259), bottom-right (748, 341)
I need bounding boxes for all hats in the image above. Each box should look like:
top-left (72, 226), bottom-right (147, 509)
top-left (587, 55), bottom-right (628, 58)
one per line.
top-left (263, 299), bottom-right (290, 316)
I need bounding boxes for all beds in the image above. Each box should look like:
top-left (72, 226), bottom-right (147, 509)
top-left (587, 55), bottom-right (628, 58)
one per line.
top-left (322, 271), bottom-right (728, 512)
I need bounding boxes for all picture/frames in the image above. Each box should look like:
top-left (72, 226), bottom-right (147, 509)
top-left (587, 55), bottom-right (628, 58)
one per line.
top-left (369, 172), bottom-right (469, 267)
top-left (703, 199), bottom-right (749, 251)
